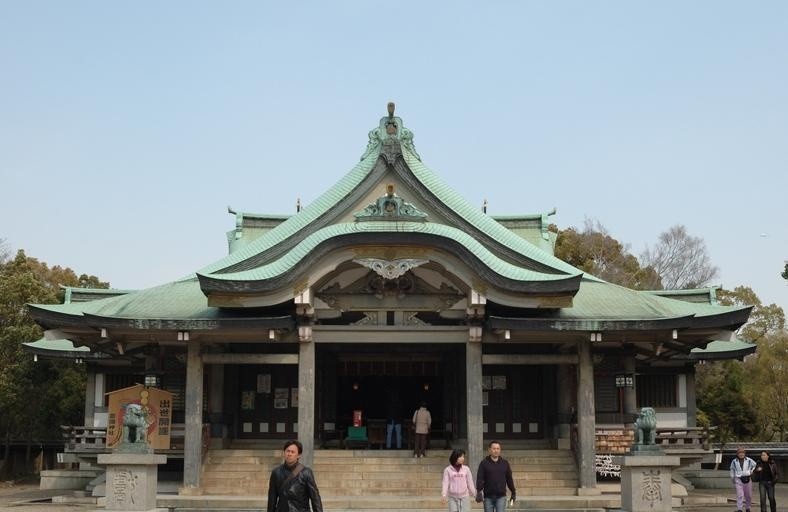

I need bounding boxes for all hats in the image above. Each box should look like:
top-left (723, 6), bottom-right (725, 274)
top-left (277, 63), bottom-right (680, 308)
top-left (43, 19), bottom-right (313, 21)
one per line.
top-left (737, 448), bottom-right (746, 454)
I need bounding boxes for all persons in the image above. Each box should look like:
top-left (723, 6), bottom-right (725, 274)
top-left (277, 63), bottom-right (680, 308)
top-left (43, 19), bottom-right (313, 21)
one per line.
top-left (385, 410), bottom-right (403, 449)
top-left (475, 440), bottom-right (516, 512)
top-left (411, 400), bottom-right (432, 458)
top-left (753, 451), bottom-right (779, 512)
top-left (267, 441), bottom-right (323, 512)
top-left (729, 448), bottom-right (757, 512)
top-left (441, 449), bottom-right (485, 512)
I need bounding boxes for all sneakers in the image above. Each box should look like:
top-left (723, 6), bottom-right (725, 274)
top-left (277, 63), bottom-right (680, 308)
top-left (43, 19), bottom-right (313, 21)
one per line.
top-left (746, 509), bottom-right (750, 512)
top-left (735, 510), bottom-right (742, 512)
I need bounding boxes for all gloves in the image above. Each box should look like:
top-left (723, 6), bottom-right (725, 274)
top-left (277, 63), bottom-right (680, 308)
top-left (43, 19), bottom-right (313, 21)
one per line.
top-left (510, 494), bottom-right (515, 503)
top-left (476, 493), bottom-right (483, 502)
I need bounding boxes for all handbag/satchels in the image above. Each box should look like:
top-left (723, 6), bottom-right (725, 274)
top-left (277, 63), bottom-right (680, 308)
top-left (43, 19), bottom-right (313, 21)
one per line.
top-left (739, 475), bottom-right (750, 483)
